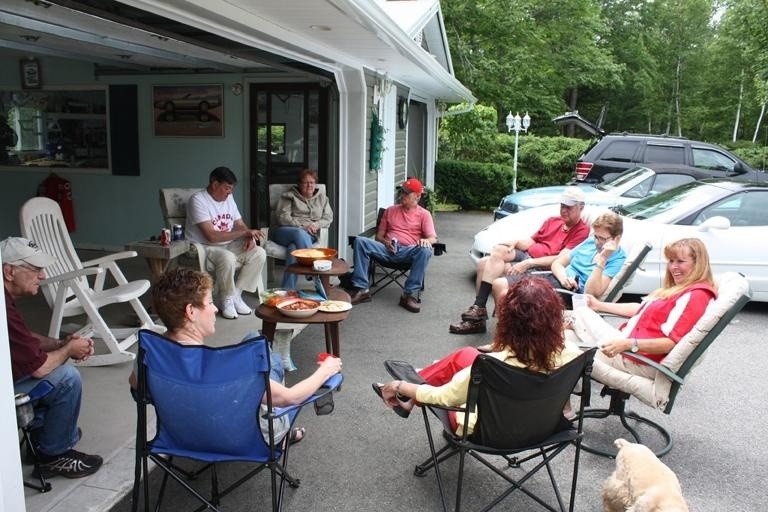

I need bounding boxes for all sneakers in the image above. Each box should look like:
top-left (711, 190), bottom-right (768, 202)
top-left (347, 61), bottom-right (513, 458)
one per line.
top-left (27, 428), bottom-right (82, 464)
top-left (32, 450), bottom-right (102, 479)
top-left (219, 294), bottom-right (237, 318)
top-left (233, 295), bottom-right (251, 314)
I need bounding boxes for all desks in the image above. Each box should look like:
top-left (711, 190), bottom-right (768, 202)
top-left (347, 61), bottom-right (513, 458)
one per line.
top-left (278, 252), bottom-right (347, 296)
top-left (255, 284), bottom-right (353, 392)
top-left (121, 234), bottom-right (190, 285)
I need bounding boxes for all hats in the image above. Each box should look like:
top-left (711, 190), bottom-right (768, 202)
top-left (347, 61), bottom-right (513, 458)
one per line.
top-left (0, 236), bottom-right (56, 268)
top-left (397, 179), bottom-right (422, 195)
top-left (557, 186), bottom-right (584, 206)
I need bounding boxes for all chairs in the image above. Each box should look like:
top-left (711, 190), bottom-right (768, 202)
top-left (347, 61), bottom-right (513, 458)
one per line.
top-left (345, 205), bottom-right (435, 299)
top-left (484, 242), bottom-right (654, 324)
top-left (124, 326), bottom-right (344, 509)
top-left (262, 181), bottom-right (334, 289)
top-left (158, 183), bottom-right (266, 314)
top-left (553, 268), bottom-right (755, 464)
top-left (17, 195), bottom-right (170, 369)
top-left (15, 375), bottom-right (58, 497)
top-left (377, 345), bottom-right (603, 509)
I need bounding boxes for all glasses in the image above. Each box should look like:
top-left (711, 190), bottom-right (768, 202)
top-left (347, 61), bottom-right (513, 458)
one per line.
top-left (590, 230), bottom-right (612, 244)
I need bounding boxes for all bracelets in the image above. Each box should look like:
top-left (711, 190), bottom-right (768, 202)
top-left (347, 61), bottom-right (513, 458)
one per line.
top-left (396, 379), bottom-right (405, 397)
top-left (596, 262), bottom-right (605, 274)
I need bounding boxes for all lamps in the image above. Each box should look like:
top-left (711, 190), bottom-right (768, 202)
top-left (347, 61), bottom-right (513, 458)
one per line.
top-left (230, 82), bottom-right (244, 99)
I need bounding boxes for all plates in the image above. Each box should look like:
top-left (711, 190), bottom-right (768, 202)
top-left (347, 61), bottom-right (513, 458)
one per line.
top-left (319, 299), bottom-right (353, 313)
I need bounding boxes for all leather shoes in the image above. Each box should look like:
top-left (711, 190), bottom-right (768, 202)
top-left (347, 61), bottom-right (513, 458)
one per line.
top-left (462, 304), bottom-right (488, 319)
top-left (351, 288), bottom-right (370, 304)
top-left (373, 381), bottom-right (413, 418)
top-left (449, 320), bottom-right (487, 334)
top-left (400, 294), bottom-right (420, 313)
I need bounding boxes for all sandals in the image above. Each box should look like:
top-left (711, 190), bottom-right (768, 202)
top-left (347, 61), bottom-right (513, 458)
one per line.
top-left (289, 427), bottom-right (304, 443)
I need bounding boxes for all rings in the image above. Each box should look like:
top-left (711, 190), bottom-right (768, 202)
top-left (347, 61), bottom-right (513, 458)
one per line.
top-left (257, 234), bottom-right (259, 237)
top-left (606, 243), bottom-right (611, 247)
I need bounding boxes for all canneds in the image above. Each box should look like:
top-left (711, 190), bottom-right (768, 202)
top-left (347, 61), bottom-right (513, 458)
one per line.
top-left (161, 228), bottom-right (171, 248)
top-left (174, 224), bottom-right (183, 241)
top-left (391, 238), bottom-right (399, 254)
top-left (14, 393), bottom-right (36, 428)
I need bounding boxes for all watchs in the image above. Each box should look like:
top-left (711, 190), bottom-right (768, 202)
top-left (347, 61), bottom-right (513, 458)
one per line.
top-left (630, 334), bottom-right (638, 354)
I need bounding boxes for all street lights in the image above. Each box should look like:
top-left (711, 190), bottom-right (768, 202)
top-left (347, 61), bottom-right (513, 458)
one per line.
top-left (504, 109), bottom-right (533, 192)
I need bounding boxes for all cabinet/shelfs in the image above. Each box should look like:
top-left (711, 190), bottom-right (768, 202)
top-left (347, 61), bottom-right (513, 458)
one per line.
top-left (0, 84), bottom-right (139, 178)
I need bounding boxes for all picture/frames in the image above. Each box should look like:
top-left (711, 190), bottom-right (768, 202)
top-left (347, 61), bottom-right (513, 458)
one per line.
top-left (19, 56), bottom-right (43, 92)
top-left (398, 95), bottom-right (410, 131)
top-left (148, 81), bottom-right (225, 139)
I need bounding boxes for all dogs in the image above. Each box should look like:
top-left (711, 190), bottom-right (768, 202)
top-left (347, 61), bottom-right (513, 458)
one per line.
top-left (602, 438), bottom-right (689, 512)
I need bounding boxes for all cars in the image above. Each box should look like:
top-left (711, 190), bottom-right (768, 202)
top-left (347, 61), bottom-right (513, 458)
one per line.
top-left (484, 162), bottom-right (716, 226)
top-left (464, 170), bottom-right (767, 311)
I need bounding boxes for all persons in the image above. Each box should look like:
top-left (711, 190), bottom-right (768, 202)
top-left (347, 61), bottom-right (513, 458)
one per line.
top-left (370, 275), bottom-right (577, 446)
top-left (561, 237), bottom-right (717, 420)
top-left (349, 178), bottom-right (439, 313)
top-left (474, 214), bottom-right (623, 352)
top-left (182, 168), bottom-right (266, 318)
top-left (1, 236), bottom-right (102, 478)
top-left (451, 190), bottom-right (588, 334)
top-left (127, 268), bottom-right (344, 449)
top-left (268, 168), bottom-right (334, 298)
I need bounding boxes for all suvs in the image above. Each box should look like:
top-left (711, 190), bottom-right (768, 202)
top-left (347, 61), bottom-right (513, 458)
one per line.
top-left (550, 109), bottom-right (768, 183)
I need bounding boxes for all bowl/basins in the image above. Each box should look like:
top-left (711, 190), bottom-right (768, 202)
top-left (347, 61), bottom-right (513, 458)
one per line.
top-left (274, 298), bottom-right (321, 318)
top-left (260, 287), bottom-right (298, 307)
top-left (288, 247), bottom-right (339, 267)
top-left (312, 260), bottom-right (334, 272)
